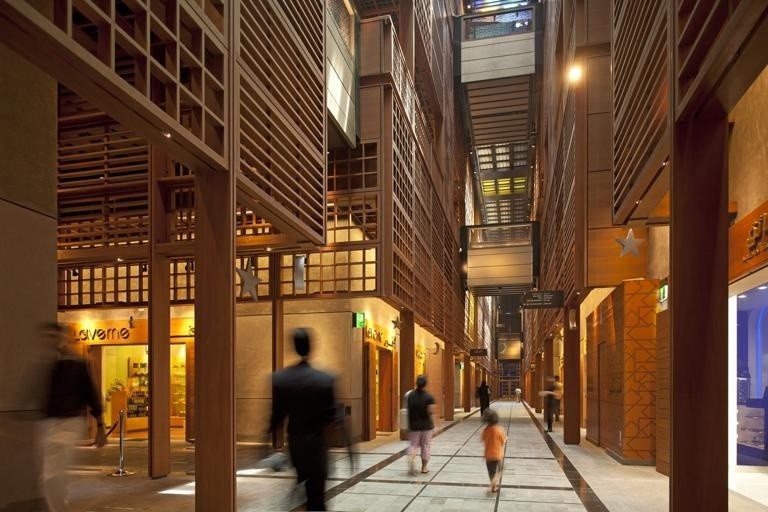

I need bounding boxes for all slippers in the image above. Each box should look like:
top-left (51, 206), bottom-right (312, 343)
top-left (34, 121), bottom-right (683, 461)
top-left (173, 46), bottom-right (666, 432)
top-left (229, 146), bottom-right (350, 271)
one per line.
top-left (421, 469), bottom-right (430, 473)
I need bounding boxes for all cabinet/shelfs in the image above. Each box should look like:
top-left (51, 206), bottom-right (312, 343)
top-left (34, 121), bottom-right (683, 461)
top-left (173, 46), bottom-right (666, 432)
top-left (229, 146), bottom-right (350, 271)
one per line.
top-left (109, 390), bottom-right (149, 440)
top-left (169, 358), bottom-right (187, 430)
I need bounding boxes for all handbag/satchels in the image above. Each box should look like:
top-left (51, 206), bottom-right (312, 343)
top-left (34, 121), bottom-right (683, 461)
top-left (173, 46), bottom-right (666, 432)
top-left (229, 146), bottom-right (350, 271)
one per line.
top-left (398, 389), bottom-right (412, 431)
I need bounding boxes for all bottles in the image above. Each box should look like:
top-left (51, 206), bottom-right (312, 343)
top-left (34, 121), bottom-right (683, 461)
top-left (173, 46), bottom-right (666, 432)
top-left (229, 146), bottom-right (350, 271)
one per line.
top-left (128, 390), bottom-right (148, 417)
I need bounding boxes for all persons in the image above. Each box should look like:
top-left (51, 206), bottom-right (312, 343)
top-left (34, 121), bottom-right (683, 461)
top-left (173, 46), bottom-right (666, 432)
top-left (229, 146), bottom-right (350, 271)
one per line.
top-left (537, 375), bottom-right (560, 432)
top-left (406, 375), bottom-right (436, 475)
top-left (21, 323), bottom-right (107, 512)
top-left (481, 412), bottom-right (506, 492)
top-left (552, 376), bottom-right (563, 424)
top-left (477, 380), bottom-right (489, 418)
top-left (268, 327), bottom-right (335, 512)
top-left (513, 387), bottom-right (522, 402)
